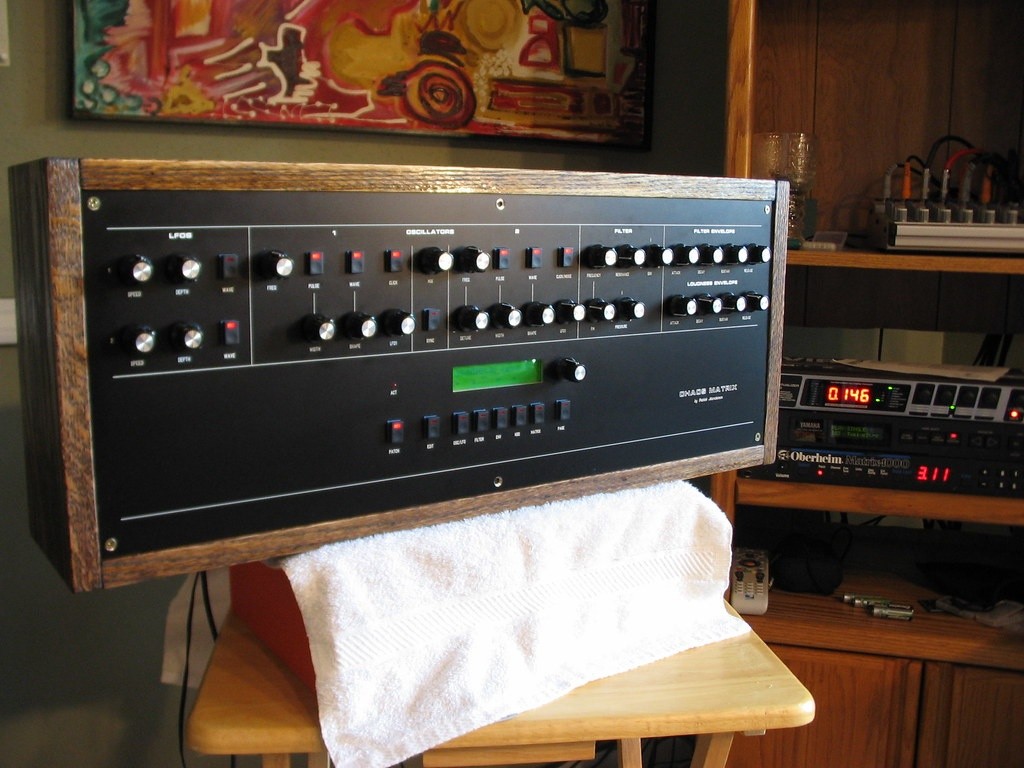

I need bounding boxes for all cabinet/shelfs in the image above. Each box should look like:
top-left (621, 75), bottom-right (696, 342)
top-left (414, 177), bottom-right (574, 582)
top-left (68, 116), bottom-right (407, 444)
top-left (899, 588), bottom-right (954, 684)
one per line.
top-left (691, 1), bottom-right (1023, 768)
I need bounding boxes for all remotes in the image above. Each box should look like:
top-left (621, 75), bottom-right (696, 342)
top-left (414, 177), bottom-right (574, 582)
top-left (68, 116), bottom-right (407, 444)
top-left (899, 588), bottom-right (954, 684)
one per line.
top-left (730, 547), bottom-right (769, 615)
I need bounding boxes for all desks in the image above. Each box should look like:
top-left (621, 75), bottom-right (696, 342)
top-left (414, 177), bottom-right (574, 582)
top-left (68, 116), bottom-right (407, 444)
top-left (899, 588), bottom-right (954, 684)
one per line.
top-left (185, 606), bottom-right (817, 768)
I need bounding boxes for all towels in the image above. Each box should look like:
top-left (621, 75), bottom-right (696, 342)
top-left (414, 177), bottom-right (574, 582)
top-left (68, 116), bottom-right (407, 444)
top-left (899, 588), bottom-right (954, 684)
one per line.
top-left (160, 478), bottom-right (752, 768)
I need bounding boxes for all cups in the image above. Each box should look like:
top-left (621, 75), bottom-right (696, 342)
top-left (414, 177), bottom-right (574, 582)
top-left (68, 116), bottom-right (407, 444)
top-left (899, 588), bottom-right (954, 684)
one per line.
top-left (759, 130), bottom-right (816, 251)
